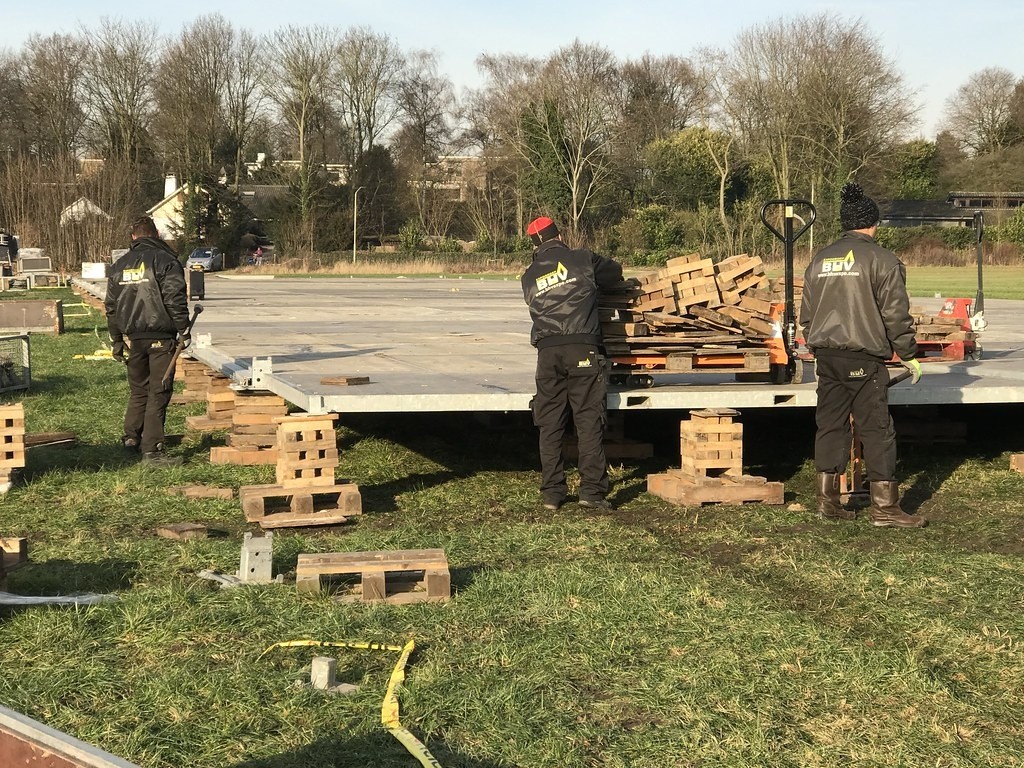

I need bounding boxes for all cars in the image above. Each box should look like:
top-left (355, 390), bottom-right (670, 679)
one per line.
top-left (186, 247), bottom-right (222, 272)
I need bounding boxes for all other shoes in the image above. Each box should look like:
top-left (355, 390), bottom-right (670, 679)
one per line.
top-left (143, 442), bottom-right (166, 461)
top-left (543, 494), bottom-right (567, 510)
top-left (578, 498), bottom-right (613, 509)
top-left (124, 436), bottom-right (140, 450)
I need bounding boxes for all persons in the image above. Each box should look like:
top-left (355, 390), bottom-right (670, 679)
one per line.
top-left (104, 216), bottom-right (191, 467)
top-left (799, 182), bottom-right (927, 528)
top-left (520, 216), bottom-right (623, 511)
top-left (255, 246), bottom-right (262, 266)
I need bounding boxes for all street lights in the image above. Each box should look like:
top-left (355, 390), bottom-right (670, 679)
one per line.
top-left (353, 186), bottom-right (367, 262)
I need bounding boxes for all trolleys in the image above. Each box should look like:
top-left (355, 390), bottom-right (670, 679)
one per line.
top-left (817, 209), bottom-right (988, 366)
top-left (608, 200), bottom-right (817, 388)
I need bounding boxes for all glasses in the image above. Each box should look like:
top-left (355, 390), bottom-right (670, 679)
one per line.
top-left (876, 220), bottom-right (882, 227)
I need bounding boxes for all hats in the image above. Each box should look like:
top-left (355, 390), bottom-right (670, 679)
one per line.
top-left (527, 217), bottom-right (559, 245)
top-left (840, 182), bottom-right (879, 230)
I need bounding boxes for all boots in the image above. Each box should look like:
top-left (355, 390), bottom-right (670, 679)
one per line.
top-left (869, 480), bottom-right (928, 528)
top-left (816, 472), bottom-right (857, 522)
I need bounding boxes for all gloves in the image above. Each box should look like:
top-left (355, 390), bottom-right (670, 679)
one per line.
top-left (112, 341), bottom-right (130, 362)
top-left (901, 358), bottom-right (922, 384)
top-left (176, 327), bottom-right (191, 350)
